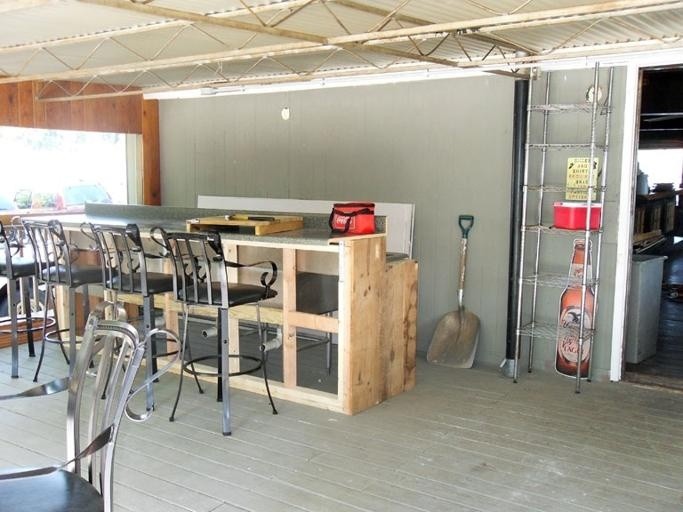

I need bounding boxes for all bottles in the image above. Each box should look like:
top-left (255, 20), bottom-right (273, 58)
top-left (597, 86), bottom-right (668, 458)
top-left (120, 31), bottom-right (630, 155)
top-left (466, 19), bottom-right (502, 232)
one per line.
top-left (637, 172), bottom-right (648, 196)
top-left (556, 238), bottom-right (594, 377)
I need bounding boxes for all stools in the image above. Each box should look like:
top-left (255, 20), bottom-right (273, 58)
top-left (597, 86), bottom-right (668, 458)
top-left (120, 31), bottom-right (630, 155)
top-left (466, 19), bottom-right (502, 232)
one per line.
top-left (150, 225), bottom-right (278, 436)
top-left (0, 218), bottom-right (66, 379)
top-left (13, 214), bottom-right (136, 395)
top-left (78, 221), bottom-right (204, 410)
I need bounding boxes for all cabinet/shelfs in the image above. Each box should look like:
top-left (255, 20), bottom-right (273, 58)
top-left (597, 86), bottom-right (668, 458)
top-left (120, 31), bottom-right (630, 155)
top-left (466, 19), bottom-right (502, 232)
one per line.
top-left (61, 214), bottom-right (420, 416)
top-left (512, 62), bottom-right (614, 396)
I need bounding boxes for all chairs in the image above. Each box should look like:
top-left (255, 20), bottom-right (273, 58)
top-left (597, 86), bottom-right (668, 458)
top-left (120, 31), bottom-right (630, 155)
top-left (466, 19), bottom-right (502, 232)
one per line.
top-left (0, 312), bottom-right (146, 511)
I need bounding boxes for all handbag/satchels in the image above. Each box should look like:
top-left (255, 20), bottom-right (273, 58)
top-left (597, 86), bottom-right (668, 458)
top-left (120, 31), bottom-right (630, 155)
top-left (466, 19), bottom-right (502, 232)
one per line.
top-left (329, 203), bottom-right (375, 234)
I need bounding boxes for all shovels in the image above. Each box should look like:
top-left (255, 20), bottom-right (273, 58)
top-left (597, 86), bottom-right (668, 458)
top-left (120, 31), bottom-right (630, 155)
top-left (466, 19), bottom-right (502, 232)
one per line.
top-left (426, 215), bottom-right (481, 368)
